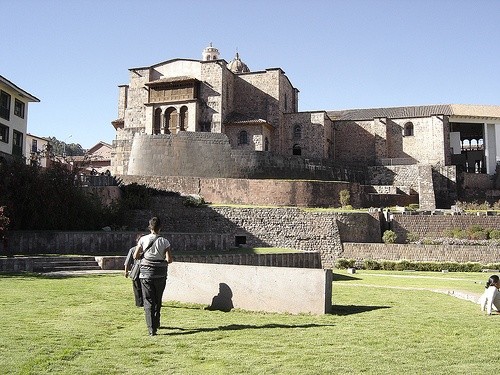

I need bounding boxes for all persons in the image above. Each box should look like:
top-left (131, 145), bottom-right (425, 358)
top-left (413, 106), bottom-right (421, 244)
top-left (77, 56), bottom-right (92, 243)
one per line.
top-left (480, 276), bottom-right (500, 317)
top-left (124, 232), bottom-right (146, 309)
top-left (134, 216), bottom-right (172, 337)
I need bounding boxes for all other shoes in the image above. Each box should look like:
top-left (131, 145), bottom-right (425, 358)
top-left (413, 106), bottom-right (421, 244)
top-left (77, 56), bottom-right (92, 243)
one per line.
top-left (156, 324), bottom-right (160, 329)
top-left (150, 332), bottom-right (157, 335)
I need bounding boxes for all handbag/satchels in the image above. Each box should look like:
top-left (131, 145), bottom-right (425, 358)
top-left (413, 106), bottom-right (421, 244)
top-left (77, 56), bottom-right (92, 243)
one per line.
top-left (129, 263), bottom-right (140, 280)
